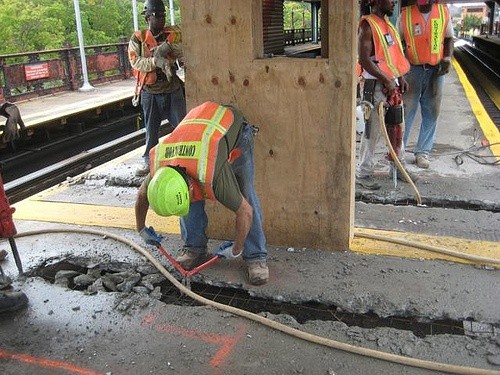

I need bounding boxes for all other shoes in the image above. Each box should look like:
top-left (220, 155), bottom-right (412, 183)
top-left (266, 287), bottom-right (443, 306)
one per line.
top-left (246, 259), bottom-right (269, 285)
top-left (355, 173), bottom-right (381, 190)
top-left (175, 248), bottom-right (208, 269)
top-left (416, 155), bottom-right (430, 168)
top-left (388, 158), bottom-right (421, 182)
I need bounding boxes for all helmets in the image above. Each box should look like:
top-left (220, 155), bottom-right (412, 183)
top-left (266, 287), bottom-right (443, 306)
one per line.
top-left (146, 166), bottom-right (189, 218)
top-left (141, 0), bottom-right (166, 16)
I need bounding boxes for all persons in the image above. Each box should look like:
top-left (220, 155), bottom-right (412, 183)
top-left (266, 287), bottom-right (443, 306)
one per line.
top-left (395, 0), bottom-right (454, 169)
top-left (356, 0), bottom-right (419, 189)
top-left (134, 101), bottom-right (269, 285)
top-left (128, 0), bottom-right (187, 176)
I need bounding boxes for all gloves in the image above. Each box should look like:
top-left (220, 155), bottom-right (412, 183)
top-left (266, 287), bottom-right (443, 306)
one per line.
top-left (215, 242), bottom-right (243, 260)
top-left (140, 226), bottom-right (162, 246)
top-left (156, 58), bottom-right (173, 82)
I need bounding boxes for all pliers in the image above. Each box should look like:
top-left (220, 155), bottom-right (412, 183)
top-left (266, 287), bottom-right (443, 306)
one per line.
top-left (144, 240), bottom-right (233, 296)
top-left (132, 71), bottom-right (148, 106)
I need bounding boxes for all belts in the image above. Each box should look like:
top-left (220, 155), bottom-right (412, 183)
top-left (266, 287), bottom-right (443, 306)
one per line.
top-left (415, 64), bottom-right (438, 71)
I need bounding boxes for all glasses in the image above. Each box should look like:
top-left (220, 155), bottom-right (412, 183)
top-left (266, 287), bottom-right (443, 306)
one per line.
top-left (151, 13), bottom-right (167, 18)
